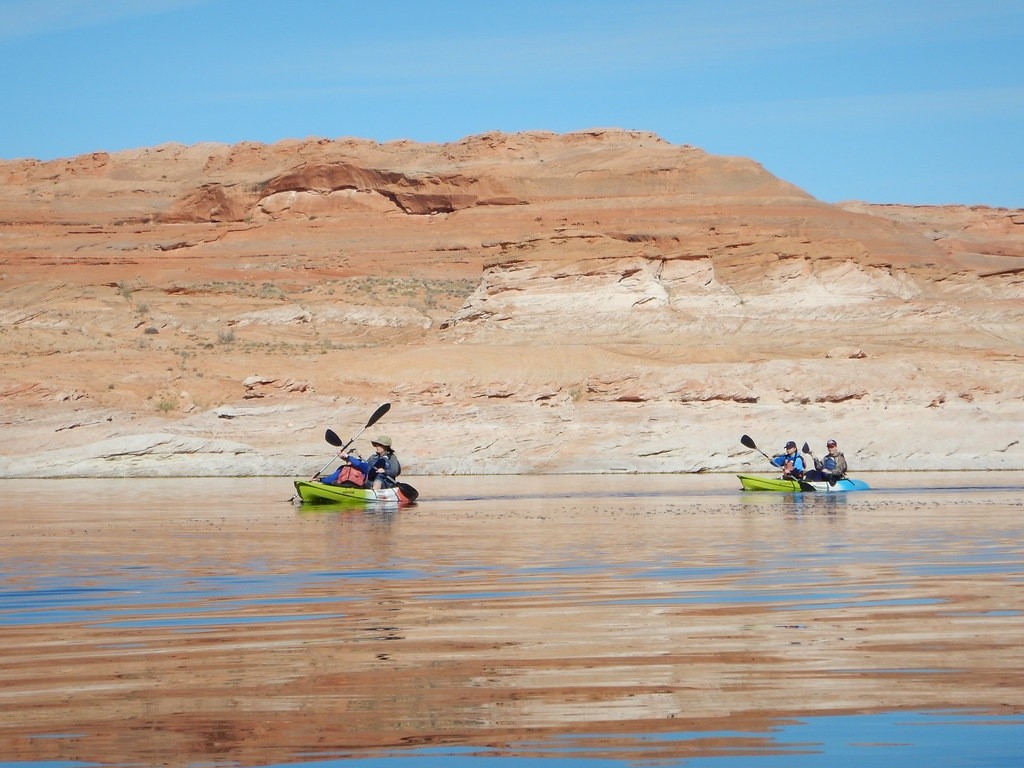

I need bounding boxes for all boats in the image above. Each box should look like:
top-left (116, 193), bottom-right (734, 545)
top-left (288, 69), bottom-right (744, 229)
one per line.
top-left (735, 473), bottom-right (872, 493)
top-left (293, 477), bottom-right (419, 506)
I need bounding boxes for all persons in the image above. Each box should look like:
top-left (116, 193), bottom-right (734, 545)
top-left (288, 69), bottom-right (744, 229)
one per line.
top-left (312, 435), bottom-right (402, 491)
top-left (768, 440), bottom-right (806, 482)
top-left (803, 438), bottom-right (848, 482)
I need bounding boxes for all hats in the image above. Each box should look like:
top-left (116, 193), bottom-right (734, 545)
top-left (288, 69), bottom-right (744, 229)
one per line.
top-left (784, 442), bottom-right (796, 448)
top-left (827, 440), bottom-right (837, 447)
top-left (371, 435), bottom-right (394, 452)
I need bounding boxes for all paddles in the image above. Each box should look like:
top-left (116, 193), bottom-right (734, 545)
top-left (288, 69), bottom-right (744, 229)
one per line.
top-left (741, 434), bottom-right (815, 491)
top-left (803, 442), bottom-right (821, 465)
top-left (324, 428), bottom-right (419, 500)
top-left (289, 402), bottom-right (391, 505)
